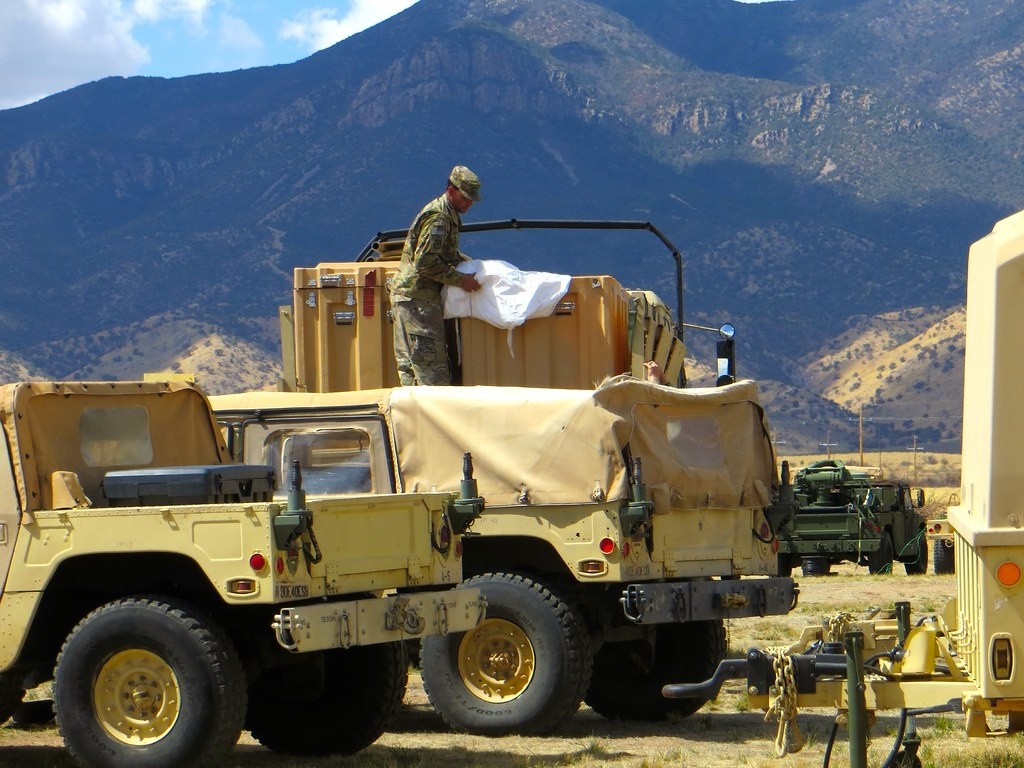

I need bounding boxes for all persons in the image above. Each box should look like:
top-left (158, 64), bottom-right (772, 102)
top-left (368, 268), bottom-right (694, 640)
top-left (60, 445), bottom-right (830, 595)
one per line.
top-left (389, 165), bottom-right (482, 386)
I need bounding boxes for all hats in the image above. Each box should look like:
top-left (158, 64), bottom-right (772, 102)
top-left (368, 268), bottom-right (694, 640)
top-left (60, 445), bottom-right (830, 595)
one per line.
top-left (450, 165), bottom-right (482, 201)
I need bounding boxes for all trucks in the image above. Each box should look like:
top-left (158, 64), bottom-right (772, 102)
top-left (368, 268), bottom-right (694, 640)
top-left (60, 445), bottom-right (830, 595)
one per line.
top-left (771, 457), bottom-right (928, 576)
top-left (143, 379), bottom-right (799, 739)
top-left (1, 371), bottom-right (489, 768)
